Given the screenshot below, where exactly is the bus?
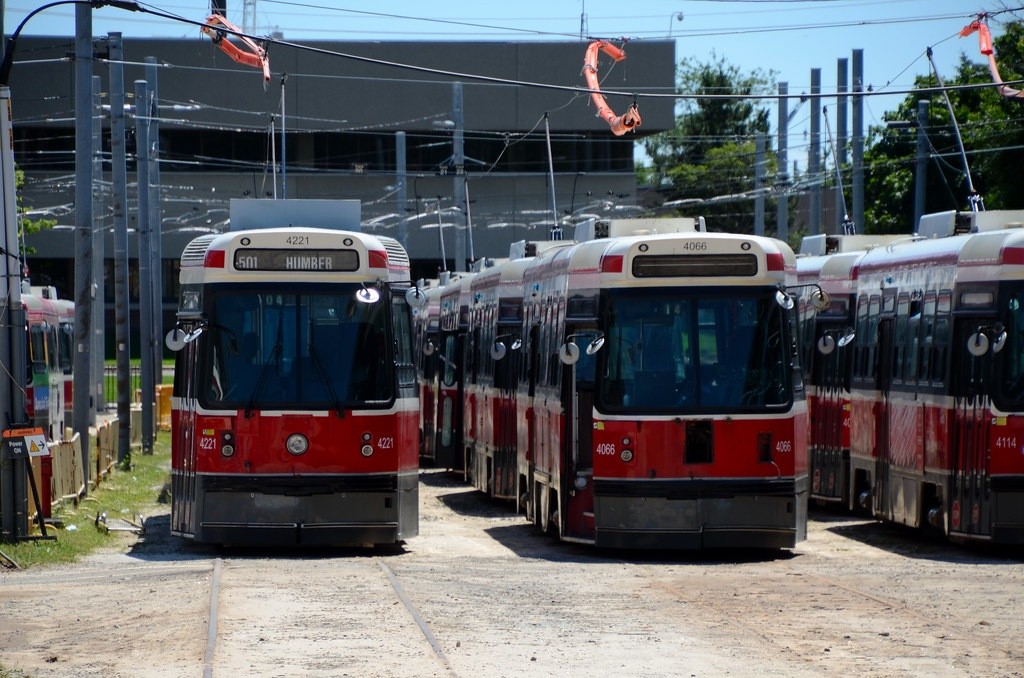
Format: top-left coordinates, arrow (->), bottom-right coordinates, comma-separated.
20,284 -> 75,418
418,47 -> 1024,549
165,74 -> 426,547
